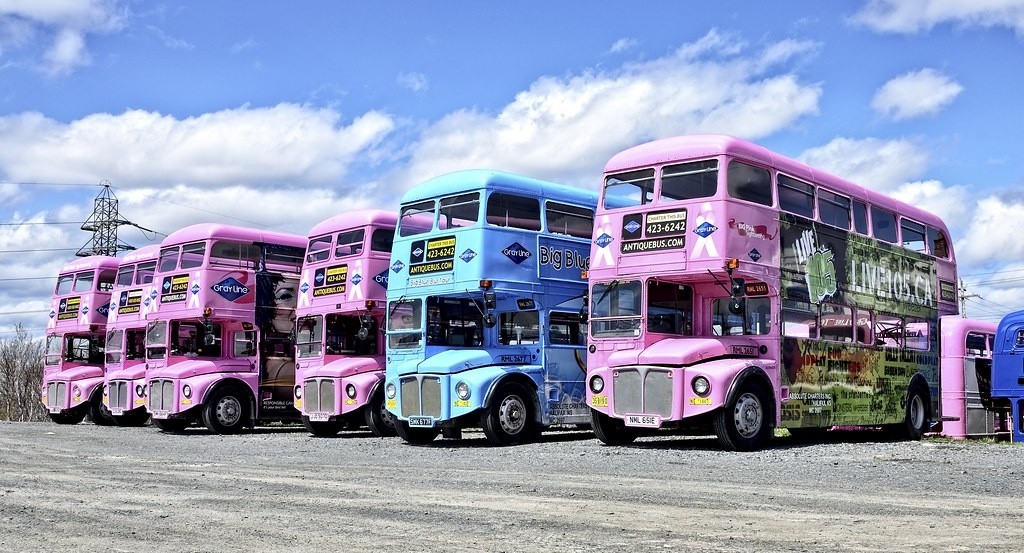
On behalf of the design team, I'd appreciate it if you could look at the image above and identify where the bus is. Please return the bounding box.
[576,133,959,453]
[141,222,391,436]
[40,255,154,427]
[91,242,298,428]
[805,308,1024,445]
[379,168,769,448]
[283,206,475,439]
